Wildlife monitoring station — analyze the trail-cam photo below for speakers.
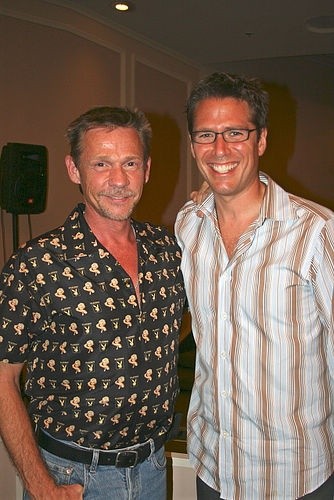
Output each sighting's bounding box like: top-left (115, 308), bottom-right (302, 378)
top-left (0, 143), bottom-right (48, 215)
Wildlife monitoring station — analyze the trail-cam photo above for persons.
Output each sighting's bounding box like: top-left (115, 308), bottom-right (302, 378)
top-left (0, 103), bottom-right (214, 500)
top-left (173, 73), bottom-right (334, 499)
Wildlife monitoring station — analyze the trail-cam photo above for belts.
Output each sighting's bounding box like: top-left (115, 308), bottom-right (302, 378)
top-left (33, 415), bottom-right (175, 469)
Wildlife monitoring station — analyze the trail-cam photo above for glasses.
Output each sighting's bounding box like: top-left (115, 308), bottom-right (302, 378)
top-left (190, 127), bottom-right (264, 145)
